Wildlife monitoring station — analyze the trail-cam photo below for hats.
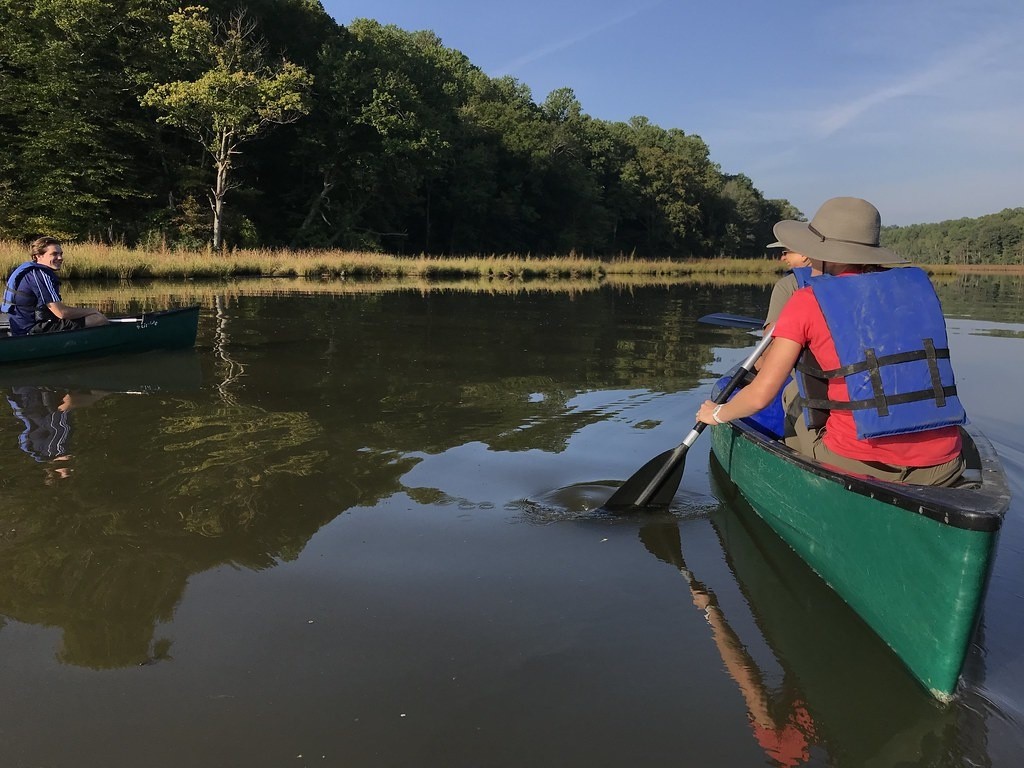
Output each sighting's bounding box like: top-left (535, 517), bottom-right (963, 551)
top-left (766, 239), bottom-right (785, 248)
top-left (773, 197), bottom-right (912, 265)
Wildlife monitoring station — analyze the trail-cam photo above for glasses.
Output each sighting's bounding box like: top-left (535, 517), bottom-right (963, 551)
top-left (782, 250), bottom-right (792, 256)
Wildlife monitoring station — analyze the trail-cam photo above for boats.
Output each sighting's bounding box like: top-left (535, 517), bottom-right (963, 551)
top-left (0, 304), bottom-right (200, 365)
top-left (715, 341), bottom-right (1013, 705)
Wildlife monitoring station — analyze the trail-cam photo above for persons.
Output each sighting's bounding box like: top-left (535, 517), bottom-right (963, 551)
top-left (4, 385), bottom-right (119, 496)
top-left (690, 579), bottom-right (836, 767)
top-left (696, 196), bottom-right (968, 488)
top-left (0, 236), bottom-right (111, 337)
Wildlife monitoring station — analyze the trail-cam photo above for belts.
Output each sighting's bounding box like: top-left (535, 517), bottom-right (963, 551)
top-left (861, 459), bottom-right (915, 474)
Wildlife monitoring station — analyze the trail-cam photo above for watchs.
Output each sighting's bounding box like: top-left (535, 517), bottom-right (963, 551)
top-left (712, 403), bottom-right (726, 423)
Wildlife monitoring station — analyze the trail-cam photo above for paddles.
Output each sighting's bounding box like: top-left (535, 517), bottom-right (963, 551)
top-left (600, 319), bottom-right (778, 512)
top-left (108, 312), bottom-right (147, 325)
